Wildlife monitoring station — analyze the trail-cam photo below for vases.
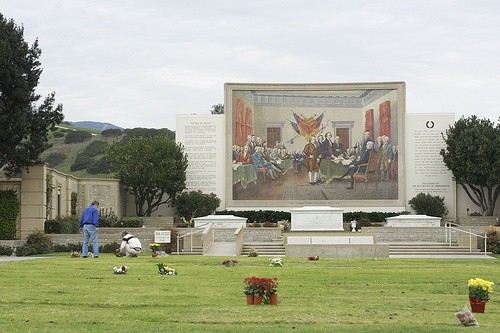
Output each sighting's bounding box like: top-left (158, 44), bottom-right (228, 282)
top-left (269, 294), bottom-right (277, 305)
top-left (152, 252), bottom-right (158, 257)
top-left (470, 299), bottom-right (485, 313)
top-left (246, 294), bottom-right (254, 304)
top-left (253, 294), bottom-right (262, 305)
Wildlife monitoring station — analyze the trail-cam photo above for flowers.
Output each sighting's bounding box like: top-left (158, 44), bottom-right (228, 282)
top-left (243, 276), bottom-right (278, 296)
top-left (222, 259), bottom-right (239, 267)
top-left (114, 266), bottom-right (127, 274)
top-left (269, 258), bottom-right (284, 267)
top-left (308, 255), bottom-right (320, 260)
top-left (468, 278), bottom-right (495, 302)
top-left (149, 244), bottom-right (160, 252)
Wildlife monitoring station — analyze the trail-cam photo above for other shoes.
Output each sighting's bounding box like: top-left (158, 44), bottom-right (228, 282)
top-left (82, 256), bottom-right (87, 258)
top-left (94, 256), bottom-right (98, 258)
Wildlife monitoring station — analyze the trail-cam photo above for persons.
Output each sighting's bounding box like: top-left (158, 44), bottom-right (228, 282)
top-left (79, 201), bottom-right (100, 258)
top-left (119, 231), bottom-right (142, 257)
